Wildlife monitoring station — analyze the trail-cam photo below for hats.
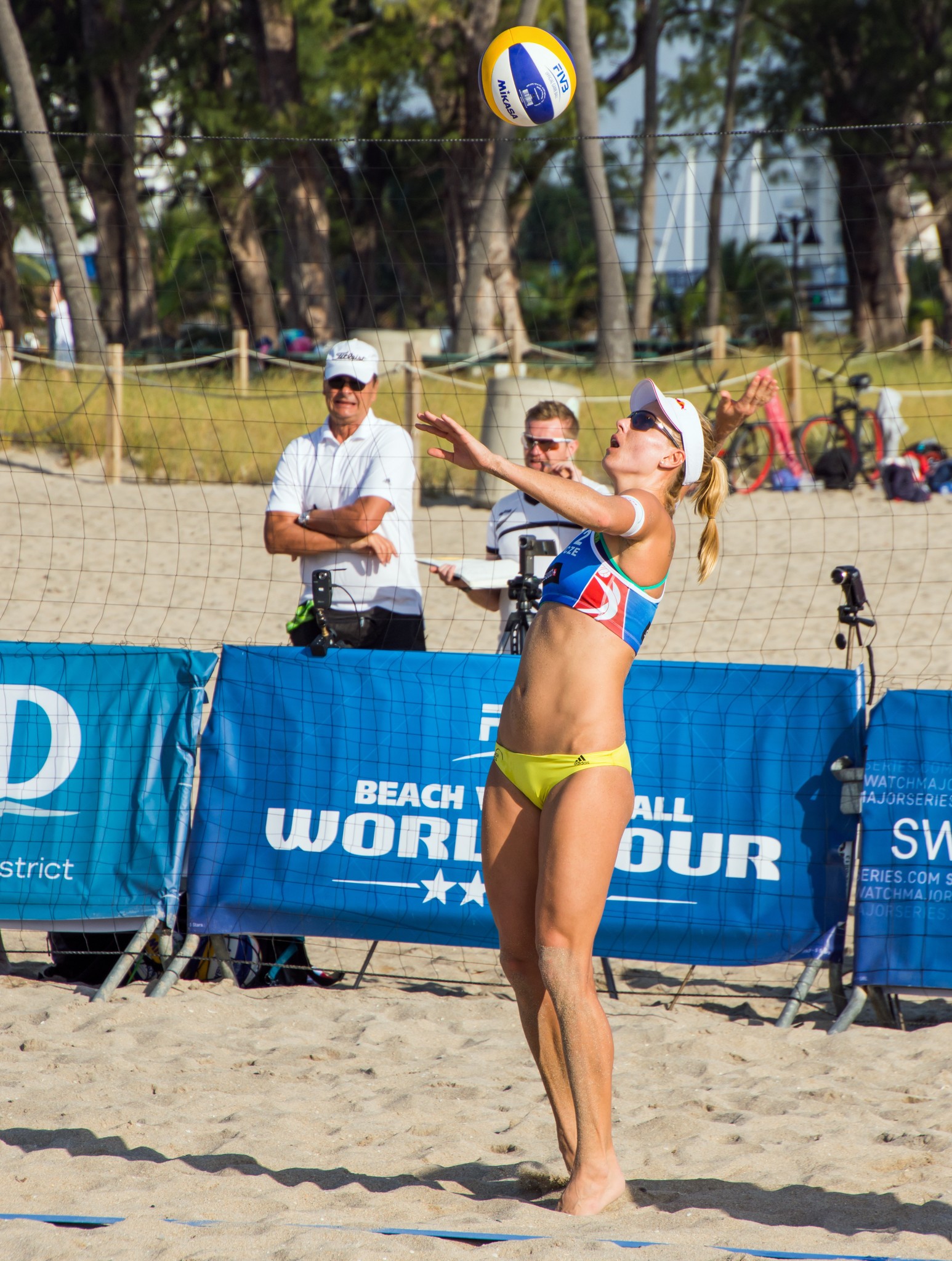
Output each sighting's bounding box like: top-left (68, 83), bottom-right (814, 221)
top-left (324, 338), bottom-right (379, 384)
top-left (630, 378), bottom-right (704, 486)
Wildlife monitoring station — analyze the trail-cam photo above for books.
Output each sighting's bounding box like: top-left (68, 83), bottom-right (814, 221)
top-left (414, 556), bottom-right (523, 591)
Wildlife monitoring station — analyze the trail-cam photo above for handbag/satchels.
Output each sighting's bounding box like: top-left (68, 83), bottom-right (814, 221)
top-left (880, 456), bottom-right (932, 503)
top-left (814, 447), bottom-right (856, 490)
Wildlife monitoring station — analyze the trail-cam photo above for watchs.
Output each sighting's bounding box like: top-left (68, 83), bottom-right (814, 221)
top-left (297, 502), bottom-right (318, 528)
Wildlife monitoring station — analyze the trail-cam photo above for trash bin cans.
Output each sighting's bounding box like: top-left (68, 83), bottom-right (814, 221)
top-left (473, 377), bottom-right (582, 510)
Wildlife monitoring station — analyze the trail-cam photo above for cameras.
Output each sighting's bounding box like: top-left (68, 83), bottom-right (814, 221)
top-left (312, 569), bottom-right (332, 610)
top-left (832, 566), bottom-right (866, 606)
top-left (518, 534), bottom-right (558, 577)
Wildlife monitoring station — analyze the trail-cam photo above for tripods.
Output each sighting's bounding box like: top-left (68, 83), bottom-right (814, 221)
top-left (497, 611), bottom-right (535, 658)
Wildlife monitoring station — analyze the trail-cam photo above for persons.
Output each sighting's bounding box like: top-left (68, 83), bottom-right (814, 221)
top-left (262, 338), bottom-right (425, 651)
top-left (431, 401), bottom-right (612, 654)
top-left (415, 371), bottom-right (781, 1207)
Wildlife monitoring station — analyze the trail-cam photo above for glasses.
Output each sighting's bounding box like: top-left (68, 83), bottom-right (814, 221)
top-left (626, 410), bottom-right (684, 469)
top-left (329, 376), bottom-right (366, 391)
top-left (521, 433), bottom-right (574, 452)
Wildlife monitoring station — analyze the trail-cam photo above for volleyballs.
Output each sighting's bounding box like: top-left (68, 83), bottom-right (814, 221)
top-left (477, 25), bottom-right (578, 128)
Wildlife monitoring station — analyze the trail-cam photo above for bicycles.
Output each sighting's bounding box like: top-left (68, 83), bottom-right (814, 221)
top-left (701, 344), bottom-right (893, 494)
